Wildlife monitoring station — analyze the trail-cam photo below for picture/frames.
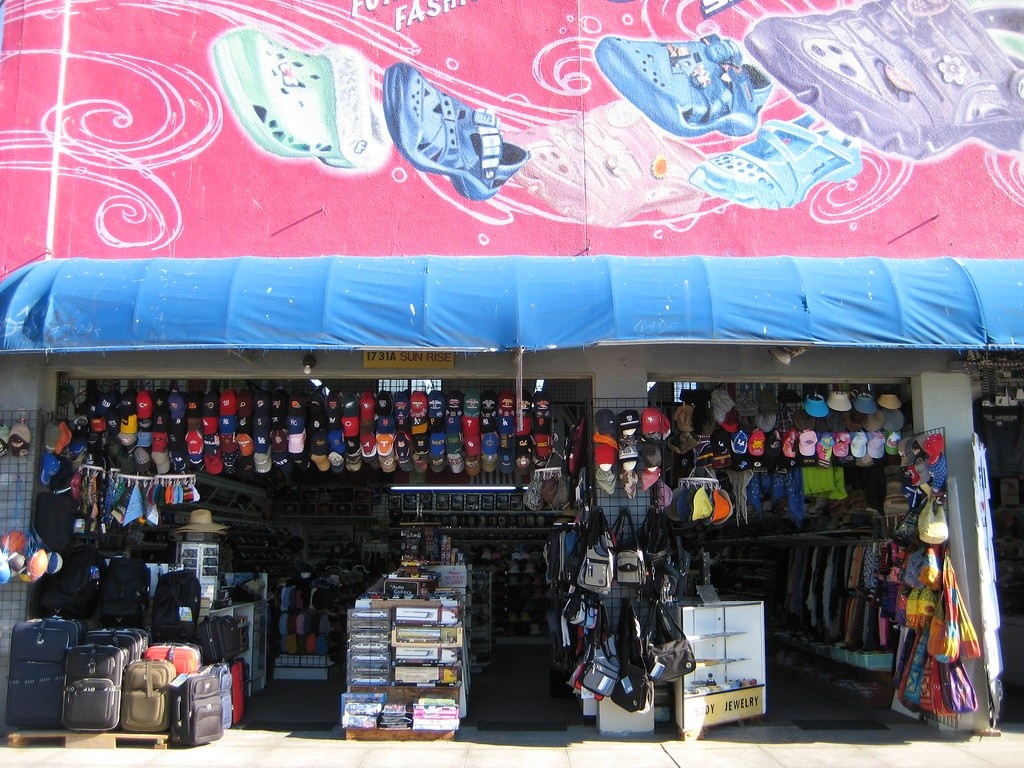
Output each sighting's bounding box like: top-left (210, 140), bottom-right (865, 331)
top-left (335, 503), bottom-right (353, 516)
top-left (281, 488), bottom-right (319, 515)
top-left (466, 494), bottom-right (479, 511)
top-left (510, 494), bottom-right (524, 511)
top-left (353, 488), bottom-right (371, 503)
top-left (388, 494), bottom-right (403, 510)
top-left (481, 494), bottom-right (495, 511)
top-left (436, 494), bottom-right (449, 511)
top-left (420, 494), bottom-right (433, 510)
top-left (451, 495), bottom-right (465, 510)
top-left (403, 494), bottom-right (417, 511)
top-left (496, 495), bottom-right (509, 511)
top-left (336, 488), bottom-right (353, 503)
top-left (354, 503), bottom-right (371, 516)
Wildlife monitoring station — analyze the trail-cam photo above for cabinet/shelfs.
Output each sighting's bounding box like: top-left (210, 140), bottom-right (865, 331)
top-left (675, 601), bottom-right (766, 728)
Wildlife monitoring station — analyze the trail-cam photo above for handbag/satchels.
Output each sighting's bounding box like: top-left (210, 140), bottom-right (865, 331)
top-left (883, 494), bottom-right (983, 715)
top-left (33, 544), bottom-right (104, 617)
top-left (150, 568), bottom-right (202, 641)
top-left (543, 507), bottom-right (698, 712)
top-left (100, 555), bottom-right (151, 627)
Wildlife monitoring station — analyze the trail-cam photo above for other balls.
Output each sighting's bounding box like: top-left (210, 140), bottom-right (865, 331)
top-left (44, 552), bottom-right (64, 574)
top-left (0, 557), bottom-right (11, 583)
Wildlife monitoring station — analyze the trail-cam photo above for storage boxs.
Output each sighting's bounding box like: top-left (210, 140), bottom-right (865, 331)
top-left (830, 647), bottom-right (839, 659)
top-left (813, 646), bottom-right (833, 654)
top-left (846, 650), bottom-right (859, 665)
top-left (857, 652), bottom-right (893, 669)
top-left (838, 648), bottom-right (849, 663)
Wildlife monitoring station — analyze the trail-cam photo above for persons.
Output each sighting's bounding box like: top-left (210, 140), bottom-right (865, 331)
top-left (280, 536), bottom-right (317, 610)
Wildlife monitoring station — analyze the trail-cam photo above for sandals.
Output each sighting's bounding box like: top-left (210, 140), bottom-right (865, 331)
top-left (384, 63), bottom-right (531, 200)
top-left (690, 112), bottom-right (864, 211)
top-left (745, 0), bottom-right (1024, 160)
top-left (597, 32), bottom-right (774, 137)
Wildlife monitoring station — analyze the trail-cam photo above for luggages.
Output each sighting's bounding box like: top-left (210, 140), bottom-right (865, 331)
top-left (4, 615), bottom-right (83, 727)
top-left (197, 610), bottom-right (242, 663)
top-left (62, 625), bottom-right (250, 747)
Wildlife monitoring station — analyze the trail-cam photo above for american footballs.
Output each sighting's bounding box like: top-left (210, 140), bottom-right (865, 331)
top-left (27, 549), bottom-right (49, 581)
top-left (8, 551), bottom-right (27, 574)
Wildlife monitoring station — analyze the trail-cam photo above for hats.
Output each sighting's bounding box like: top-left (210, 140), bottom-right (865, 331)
top-left (272, 559), bottom-right (371, 664)
top-left (473, 550), bottom-right (544, 637)
top-left (0, 383), bottom-right (947, 533)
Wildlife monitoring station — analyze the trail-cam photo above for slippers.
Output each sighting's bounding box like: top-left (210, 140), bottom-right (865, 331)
top-left (502, 95), bottom-right (707, 225)
top-left (210, 25), bottom-right (393, 170)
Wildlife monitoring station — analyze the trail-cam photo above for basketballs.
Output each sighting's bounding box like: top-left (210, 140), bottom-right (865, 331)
top-left (1, 530), bottom-right (26, 553)
